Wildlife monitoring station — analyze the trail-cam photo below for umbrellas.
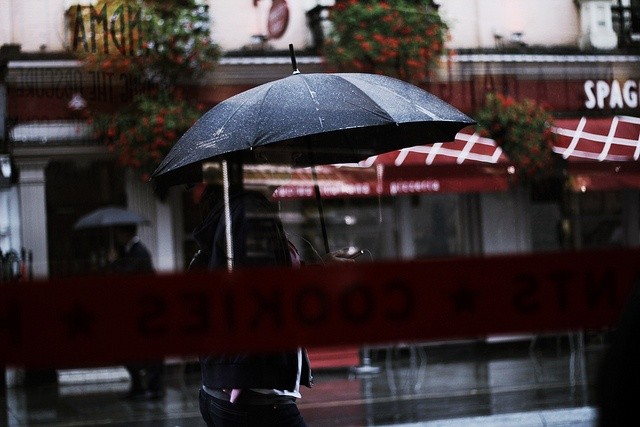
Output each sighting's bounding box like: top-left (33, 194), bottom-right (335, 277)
top-left (150, 44), bottom-right (478, 255)
top-left (72, 207), bottom-right (151, 229)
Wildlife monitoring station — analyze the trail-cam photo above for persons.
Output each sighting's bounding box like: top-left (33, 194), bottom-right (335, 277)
top-left (199, 193), bottom-right (355, 426)
top-left (107, 223), bottom-right (165, 399)
